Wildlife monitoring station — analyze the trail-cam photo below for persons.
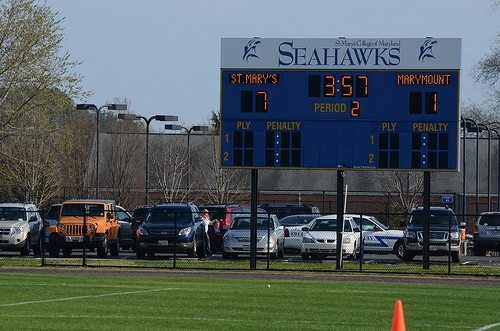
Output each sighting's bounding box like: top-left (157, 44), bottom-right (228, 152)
top-left (198, 209), bottom-right (214, 259)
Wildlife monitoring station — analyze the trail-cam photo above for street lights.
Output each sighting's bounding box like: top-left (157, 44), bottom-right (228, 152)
top-left (164, 124), bottom-right (209, 206)
top-left (75, 103), bottom-right (128, 199)
top-left (117, 114), bottom-right (178, 206)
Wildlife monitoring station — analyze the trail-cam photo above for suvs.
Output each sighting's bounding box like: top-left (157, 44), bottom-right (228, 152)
top-left (48, 199), bottom-right (122, 256)
top-left (304, 214), bottom-right (406, 259)
top-left (0, 203), bottom-right (43, 256)
top-left (473, 211), bottom-right (500, 257)
top-left (134, 202), bottom-right (205, 260)
top-left (400, 204), bottom-right (462, 263)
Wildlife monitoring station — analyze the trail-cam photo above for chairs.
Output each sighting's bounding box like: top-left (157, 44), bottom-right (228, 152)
top-left (262, 221), bottom-right (272, 226)
top-left (239, 221), bottom-right (249, 226)
top-left (318, 223), bottom-right (332, 230)
top-left (89, 207), bottom-right (98, 214)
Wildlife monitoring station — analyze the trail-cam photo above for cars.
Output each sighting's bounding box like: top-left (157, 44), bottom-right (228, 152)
top-left (279, 215), bottom-right (321, 249)
top-left (115, 205), bottom-right (134, 250)
top-left (301, 219), bottom-right (364, 260)
top-left (41, 204), bottom-right (62, 252)
top-left (223, 214), bottom-right (285, 260)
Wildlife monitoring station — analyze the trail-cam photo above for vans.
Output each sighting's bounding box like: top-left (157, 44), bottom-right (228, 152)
top-left (198, 204), bottom-right (270, 247)
